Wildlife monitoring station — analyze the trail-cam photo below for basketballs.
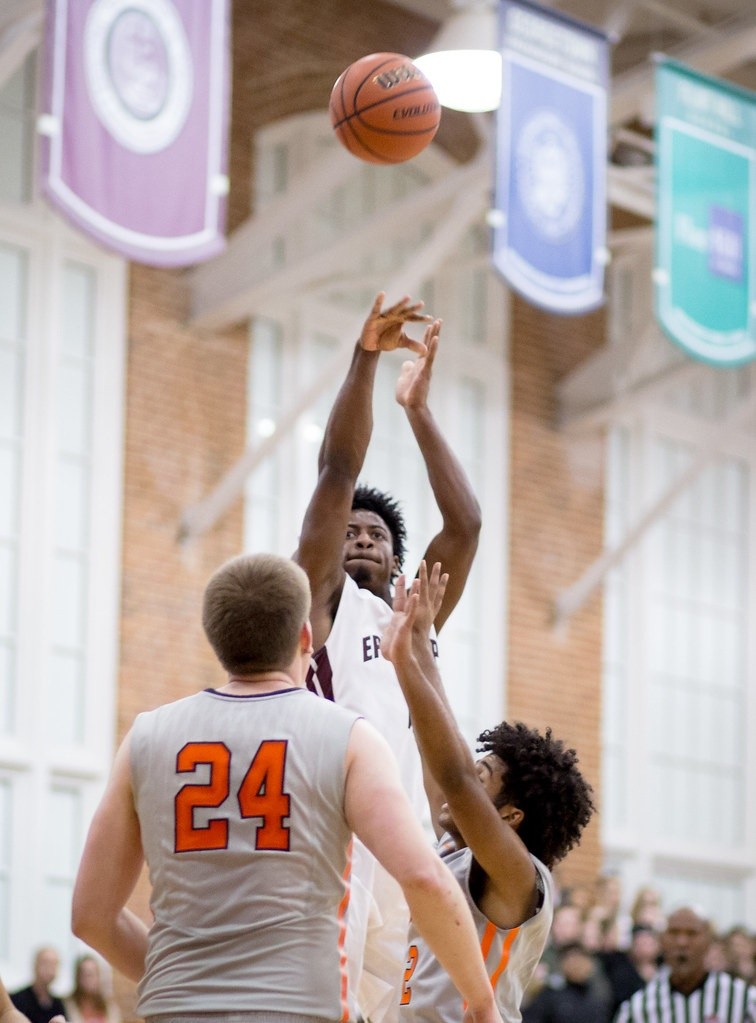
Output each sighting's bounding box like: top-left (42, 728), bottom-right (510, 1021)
top-left (328, 49), bottom-right (441, 166)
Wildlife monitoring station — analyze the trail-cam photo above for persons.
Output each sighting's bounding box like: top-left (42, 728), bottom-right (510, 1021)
top-left (71, 552), bottom-right (505, 1022)
top-left (384, 558), bottom-right (591, 1022)
top-left (2, 947), bottom-right (122, 1023)
top-left (288, 292), bottom-right (482, 1023)
top-left (523, 884), bottom-right (756, 1022)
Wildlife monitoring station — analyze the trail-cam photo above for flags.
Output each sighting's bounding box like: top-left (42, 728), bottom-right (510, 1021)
top-left (40, 1), bottom-right (234, 270)
top-left (486, 1), bottom-right (756, 367)
